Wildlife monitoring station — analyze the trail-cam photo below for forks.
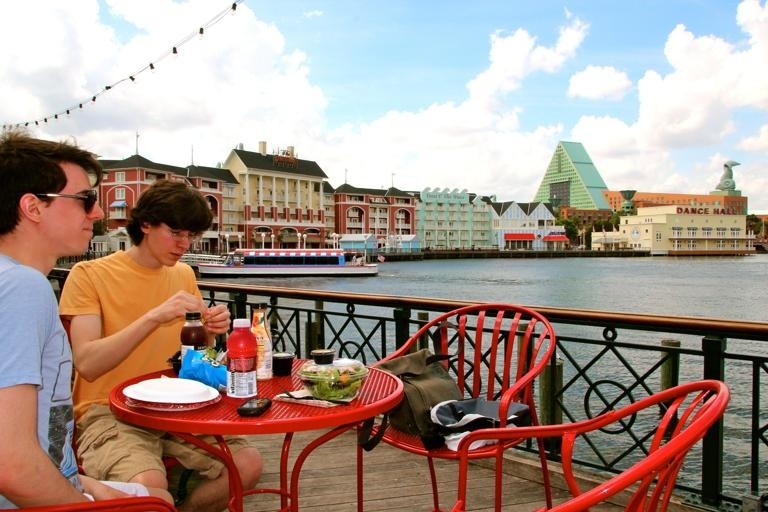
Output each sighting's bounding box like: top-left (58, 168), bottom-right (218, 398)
top-left (282, 388), bottom-right (351, 406)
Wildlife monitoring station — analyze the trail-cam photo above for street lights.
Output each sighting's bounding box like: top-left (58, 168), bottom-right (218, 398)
top-left (223, 232), bottom-right (342, 250)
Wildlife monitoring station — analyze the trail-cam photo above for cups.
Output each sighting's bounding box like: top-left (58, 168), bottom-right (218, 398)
top-left (272, 353), bottom-right (296, 376)
top-left (311, 349), bottom-right (335, 364)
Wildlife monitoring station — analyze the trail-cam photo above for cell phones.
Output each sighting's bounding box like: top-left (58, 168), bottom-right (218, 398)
top-left (237, 397), bottom-right (272, 416)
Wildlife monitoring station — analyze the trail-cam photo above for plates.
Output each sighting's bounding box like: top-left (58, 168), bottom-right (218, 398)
top-left (123, 378), bottom-right (222, 412)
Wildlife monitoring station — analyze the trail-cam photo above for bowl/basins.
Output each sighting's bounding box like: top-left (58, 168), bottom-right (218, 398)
top-left (296, 361), bottom-right (370, 398)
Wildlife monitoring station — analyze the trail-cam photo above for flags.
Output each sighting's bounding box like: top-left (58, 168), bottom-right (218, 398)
top-left (378, 254), bottom-right (385, 263)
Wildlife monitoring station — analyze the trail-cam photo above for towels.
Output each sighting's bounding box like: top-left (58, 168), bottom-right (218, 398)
top-left (273, 388), bottom-right (341, 408)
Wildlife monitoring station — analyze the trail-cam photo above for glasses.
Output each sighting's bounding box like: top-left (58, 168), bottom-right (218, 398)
top-left (36, 190), bottom-right (97, 214)
top-left (147, 227), bottom-right (203, 242)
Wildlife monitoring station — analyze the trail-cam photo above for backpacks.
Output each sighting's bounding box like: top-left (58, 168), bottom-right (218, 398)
top-left (427, 397), bottom-right (531, 451)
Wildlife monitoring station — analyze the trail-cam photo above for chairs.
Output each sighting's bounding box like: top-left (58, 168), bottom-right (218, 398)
top-left (450, 378), bottom-right (730, 512)
top-left (2, 498), bottom-right (176, 512)
top-left (58, 291), bottom-right (195, 505)
top-left (354, 303), bottom-right (557, 512)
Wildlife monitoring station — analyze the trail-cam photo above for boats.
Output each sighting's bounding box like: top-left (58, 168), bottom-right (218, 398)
top-left (196, 248), bottom-right (380, 278)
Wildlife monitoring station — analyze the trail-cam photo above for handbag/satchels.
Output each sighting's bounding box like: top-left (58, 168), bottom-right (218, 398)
top-left (358, 348), bottom-right (464, 448)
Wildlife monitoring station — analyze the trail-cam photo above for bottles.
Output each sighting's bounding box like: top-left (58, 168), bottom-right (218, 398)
top-left (226, 317), bottom-right (259, 401)
top-left (180, 311), bottom-right (206, 366)
top-left (250, 303), bottom-right (276, 381)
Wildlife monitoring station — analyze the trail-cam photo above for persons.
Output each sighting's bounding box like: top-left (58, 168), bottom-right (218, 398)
top-left (0, 134), bottom-right (175, 510)
top-left (57, 177), bottom-right (264, 511)
